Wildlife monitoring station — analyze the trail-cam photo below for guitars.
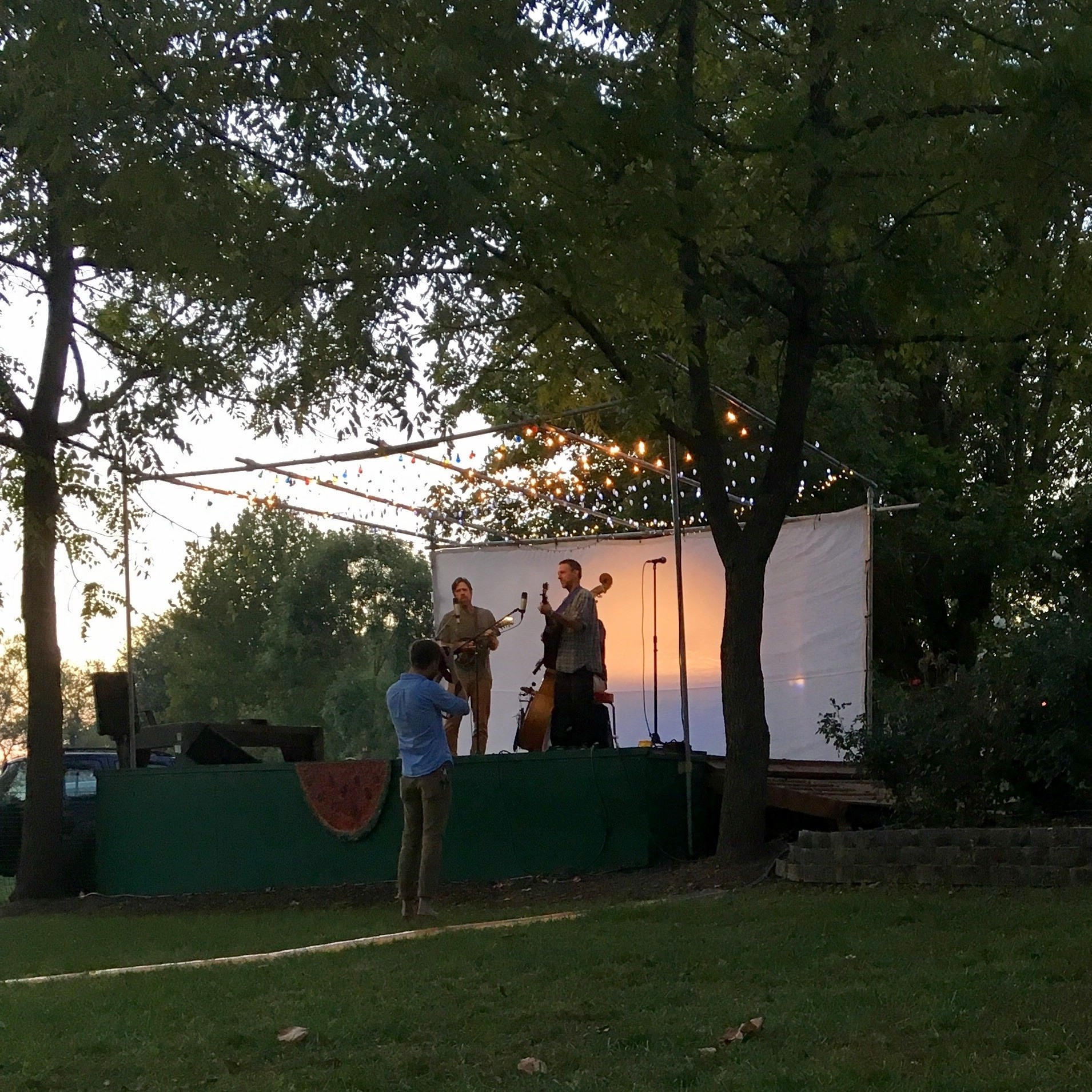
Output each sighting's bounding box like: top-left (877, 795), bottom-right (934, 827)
top-left (538, 581), bottom-right (565, 669)
top-left (454, 616), bottom-right (514, 668)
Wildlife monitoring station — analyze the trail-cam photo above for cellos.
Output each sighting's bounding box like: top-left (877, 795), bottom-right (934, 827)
top-left (517, 572), bottom-right (614, 753)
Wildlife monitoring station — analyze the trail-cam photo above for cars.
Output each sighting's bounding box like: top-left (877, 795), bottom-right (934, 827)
top-left (11, 781), bottom-right (99, 801)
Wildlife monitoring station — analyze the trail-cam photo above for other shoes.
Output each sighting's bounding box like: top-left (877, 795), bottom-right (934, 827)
top-left (418, 908), bottom-right (439, 916)
top-left (400, 910), bottom-right (413, 917)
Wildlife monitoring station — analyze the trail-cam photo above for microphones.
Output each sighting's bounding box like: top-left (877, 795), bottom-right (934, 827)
top-left (518, 592), bottom-right (528, 614)
top-left (452, 597), bottom-right (462, 624)
top-left (647, 557), bottom-right (667, 564)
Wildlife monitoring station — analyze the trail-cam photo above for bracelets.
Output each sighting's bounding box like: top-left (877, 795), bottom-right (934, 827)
top-left (546, 609), bottom-right (554, 617)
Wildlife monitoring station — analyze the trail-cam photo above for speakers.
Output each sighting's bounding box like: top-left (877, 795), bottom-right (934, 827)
top-left (185, 726), bottom-right (264, 764)
top-left (550, 705), bottom-right (613, 749)
top-left (92, 671), bottom-right (141, 735)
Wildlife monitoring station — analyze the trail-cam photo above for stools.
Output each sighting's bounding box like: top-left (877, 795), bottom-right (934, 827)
top-left (593, 692), bottom-right (619, 748)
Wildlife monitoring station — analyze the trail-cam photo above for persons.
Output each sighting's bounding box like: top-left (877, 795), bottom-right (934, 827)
top-left (434, 576), bottom-right (501, 758)
top-left (538, 559), bottom-right (603, 751)
top-left (385, 639), bottom-right (470, 917)
top-left (593, 620), bottom-right (608, 693)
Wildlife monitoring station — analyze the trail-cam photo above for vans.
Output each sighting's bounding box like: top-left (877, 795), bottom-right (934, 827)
top-left (0, 747), bottom-right (176, 878)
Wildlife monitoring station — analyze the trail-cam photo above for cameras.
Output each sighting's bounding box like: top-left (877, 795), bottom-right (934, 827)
top-left (437, 645), bottom-right (450, 671)
